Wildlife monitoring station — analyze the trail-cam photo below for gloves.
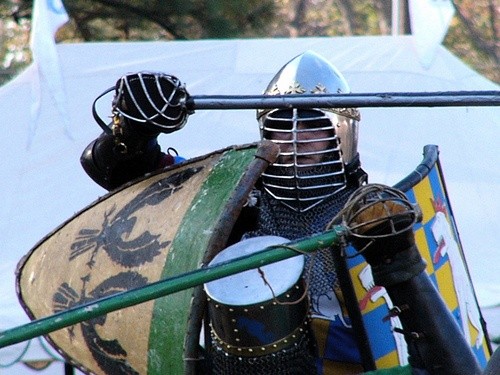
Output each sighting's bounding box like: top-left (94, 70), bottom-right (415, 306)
top-left (116, 78), bottom-right (188, 135)
top-left (345, 180), bottom-right (418, 255)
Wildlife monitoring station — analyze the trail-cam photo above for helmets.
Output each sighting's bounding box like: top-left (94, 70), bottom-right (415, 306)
top-left (256, 52), bottom-right (360, 212)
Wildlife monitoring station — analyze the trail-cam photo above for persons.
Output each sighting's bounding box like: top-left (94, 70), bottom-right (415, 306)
top-left (80, 53), bottom-right (374, 375)
top-left (341, 184), bottom-right (485, 375)
